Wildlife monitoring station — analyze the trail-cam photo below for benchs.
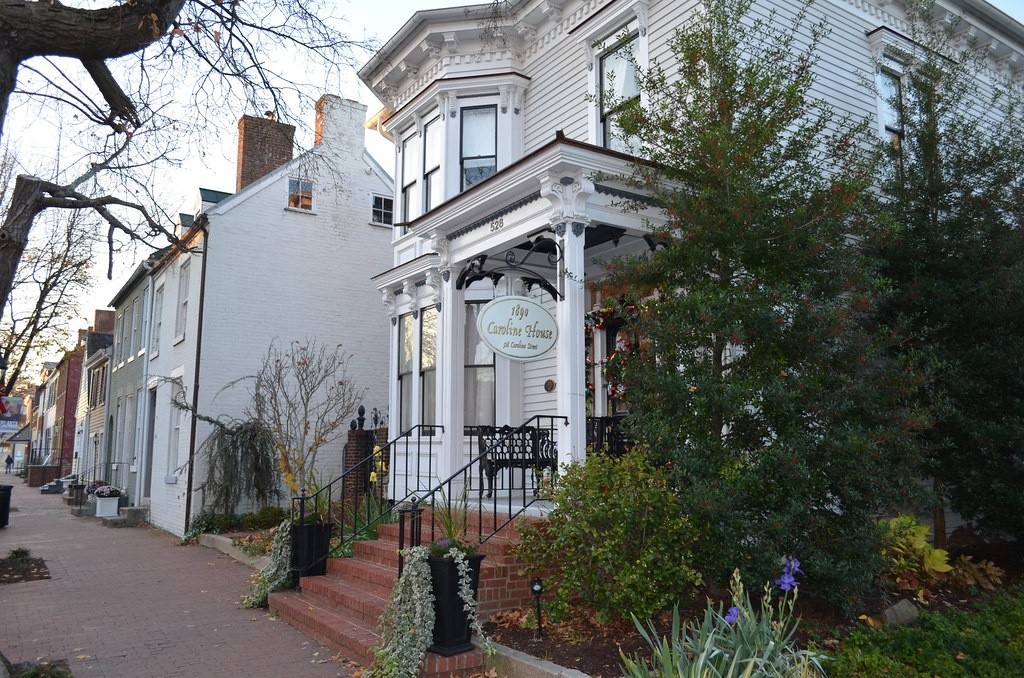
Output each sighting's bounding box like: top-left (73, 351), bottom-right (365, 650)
top-left (476, 425), bottom-right (558, 498)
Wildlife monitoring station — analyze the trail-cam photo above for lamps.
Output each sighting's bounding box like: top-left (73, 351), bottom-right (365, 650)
top-left (531, 577), bottom-right (543, 595)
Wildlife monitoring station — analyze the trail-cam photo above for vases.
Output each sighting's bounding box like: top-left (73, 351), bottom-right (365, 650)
top-left (95, 496), bottom-right (119, 518)
top-left (88, 494), bottom-right (97, 500)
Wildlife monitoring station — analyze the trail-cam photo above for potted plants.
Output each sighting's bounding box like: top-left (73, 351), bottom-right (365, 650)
top-left (242, 470), bottom-right (335, 608)
top-left (362, 476), bottom-right (497, 678)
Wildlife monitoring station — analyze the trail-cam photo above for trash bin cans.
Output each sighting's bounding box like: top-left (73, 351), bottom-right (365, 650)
top-left (0, 484), bottom-right (15, 530)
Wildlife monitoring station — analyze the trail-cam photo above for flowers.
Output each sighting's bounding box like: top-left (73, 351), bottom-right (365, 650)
top-left (303, 511), bottom-right (312, 523)
top-left (430, 536), bottom-right (454, 557)
top-left (87, 480), bottom-right (121, 498)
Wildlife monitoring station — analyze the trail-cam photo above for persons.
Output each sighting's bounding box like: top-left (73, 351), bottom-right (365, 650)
top-left (4, 455), bottom-right (14, 474)
